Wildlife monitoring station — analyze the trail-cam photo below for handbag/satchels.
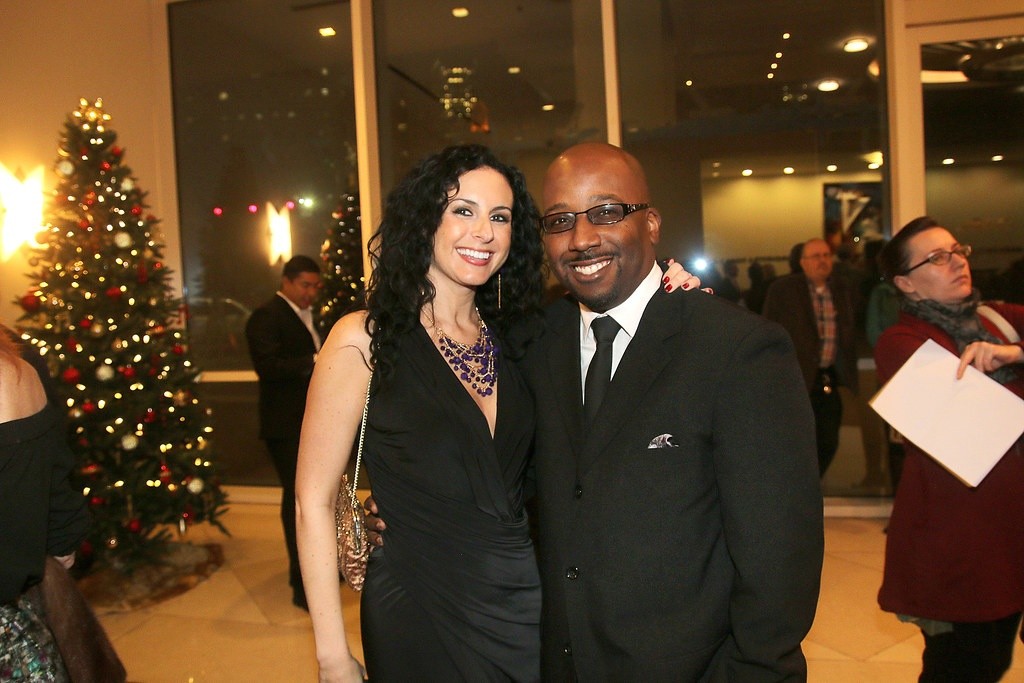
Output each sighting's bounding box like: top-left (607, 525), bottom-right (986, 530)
top-left (336, 476), bottom-right (370, 592)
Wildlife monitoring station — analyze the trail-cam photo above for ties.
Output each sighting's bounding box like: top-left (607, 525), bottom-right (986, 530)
top-left (582, 316), bottom-right (622, 432)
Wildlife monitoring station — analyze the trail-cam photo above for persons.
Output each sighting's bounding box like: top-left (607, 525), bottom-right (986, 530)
top-left (243, 257), bottom-right (351, 618)
top-left (296, 138), bottom-right (719, 682)
top-left (657, 238), bottom-right (893, 327)
top-left (764, 235), bottom-right (854, 478)
top-left (0, 332), bottom-right (122, 681)
top-left (877, 218), bottom-right (1024, 683)
top-left (356, 138), bottom-right (825, 682)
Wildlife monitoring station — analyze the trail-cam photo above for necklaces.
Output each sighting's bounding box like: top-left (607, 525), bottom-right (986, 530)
top-left (420, 300), bottom-right (500, 398)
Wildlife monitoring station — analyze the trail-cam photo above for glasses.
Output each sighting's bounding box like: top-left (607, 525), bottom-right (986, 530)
top-left (903, 244), bottom-right (972, 276)
top-left (805, 254), bottom-right (833, 260)
top-left (539, 203), bottom-right (652, 234)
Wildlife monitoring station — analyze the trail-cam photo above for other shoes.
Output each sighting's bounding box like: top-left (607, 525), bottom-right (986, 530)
top-left (292, 590), bottom-right (308, 612)
